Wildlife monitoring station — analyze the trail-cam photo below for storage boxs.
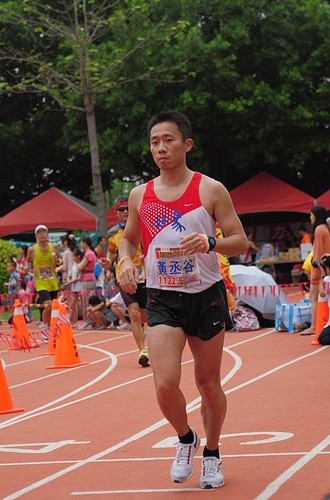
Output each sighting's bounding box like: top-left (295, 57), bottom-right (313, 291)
top-left (280, 251), bottom-right (289, 260)
top-left (288, 248), bottom-right (300, 259)
top-left (272, 270), bottom-right (302, 277)
top-left (300, 243), bottom-right (312, 259)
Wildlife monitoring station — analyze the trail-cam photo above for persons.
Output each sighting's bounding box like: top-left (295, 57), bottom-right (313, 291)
top-left (117, 109), bottom-right (250, 489)
top-left (316, 253), bottom-right (330, 346)
top-left (24, 224), bottom-right (63, 331)
top-left (99, 196), bottom-right (151, 368)
top-left (297, 225), bottom-right (311, 245)
top-left (235, 229), bottom-right (261, 263)
top-left (297, 206), bottom-right (330, 335)
top-left (3, 235), bottom-right (131, 331)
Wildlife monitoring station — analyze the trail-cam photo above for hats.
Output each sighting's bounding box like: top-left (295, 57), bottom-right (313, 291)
top-left (34, 225), bottom-right (49, 235)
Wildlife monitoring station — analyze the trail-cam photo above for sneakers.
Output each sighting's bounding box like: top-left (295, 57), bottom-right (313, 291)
top-left (198, 452), bottom-right (224, 490)
top-left (138, 350), bottom-right (150, 367)
top-left (171, 426), bottom-right (201, 483)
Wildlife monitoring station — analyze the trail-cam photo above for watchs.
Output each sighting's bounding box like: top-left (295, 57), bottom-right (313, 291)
top-left (206, 236), bottom-right (216, 255)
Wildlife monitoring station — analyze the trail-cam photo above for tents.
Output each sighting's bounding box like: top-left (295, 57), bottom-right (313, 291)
top-left (317, 190), bottom-right (330, 210)
top-left (0, 187), bottom-right (118, 235)
top-left (229, 171), bottom-right (317, 215)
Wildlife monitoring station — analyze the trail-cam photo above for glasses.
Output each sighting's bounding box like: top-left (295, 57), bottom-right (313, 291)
top-left (116, 207), bottom-right (129, 212)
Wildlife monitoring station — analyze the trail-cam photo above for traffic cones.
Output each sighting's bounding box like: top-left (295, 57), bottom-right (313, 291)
top-left (41, 299), bottom-right (61, 355)
top-left (44, 303), bottom-right (89, 369)
top-left (0, 356), bottom-right (25, 415)
top-left (6, 298), bottom-right (40, 350)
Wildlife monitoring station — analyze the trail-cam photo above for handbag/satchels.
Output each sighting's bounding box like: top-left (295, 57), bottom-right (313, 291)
top-left (275, 299), bottom-right (312, 332)
top-left (233, 305), bottom-right (260, 332)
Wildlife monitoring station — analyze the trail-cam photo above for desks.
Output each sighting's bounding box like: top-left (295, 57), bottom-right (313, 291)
top-left (253, 258), bottom-right (307, 282)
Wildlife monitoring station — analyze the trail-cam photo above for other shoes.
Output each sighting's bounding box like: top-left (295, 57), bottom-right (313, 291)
top-left (298, 328), bottom-right (315, 334)
top-left (105, 325), bottom-right (115, 330)
top-left (116, 323), bottom-right (128, 330)
top-left (97, 325), bottom-right (106, 330)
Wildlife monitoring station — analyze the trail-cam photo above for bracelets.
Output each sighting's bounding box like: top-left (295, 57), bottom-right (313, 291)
top-left (118, 256), bottom-right (132, 268)
top-left (56, 259), bottom-right (62, 265)
